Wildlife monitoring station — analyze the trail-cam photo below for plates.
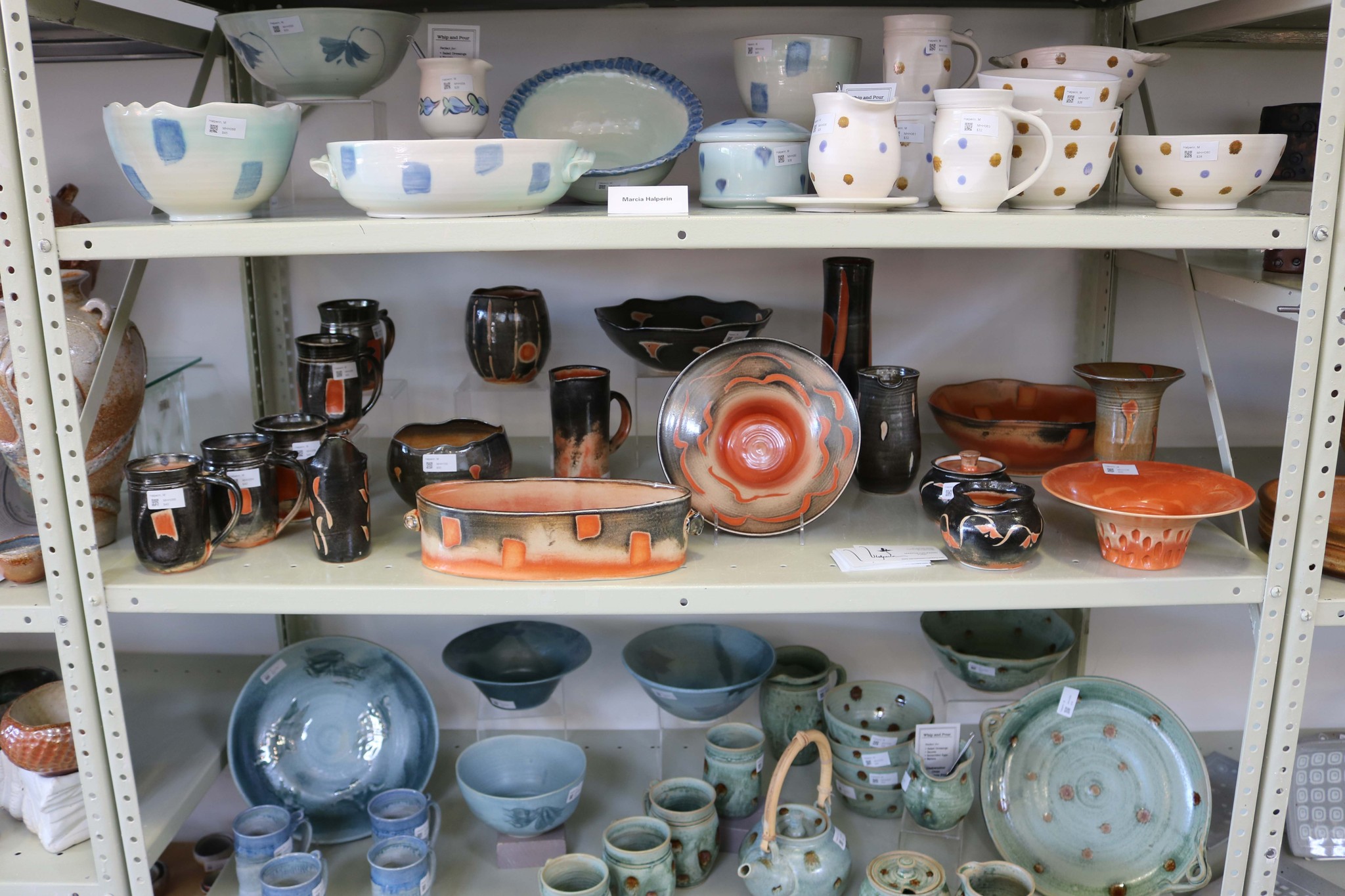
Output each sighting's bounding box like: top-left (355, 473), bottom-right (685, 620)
top-left (978, 676), bottom-right (1345, 896)
top-left (227, 634), bottom-right (440, 845)
top-left (656, 337), bottom-right (862, 540)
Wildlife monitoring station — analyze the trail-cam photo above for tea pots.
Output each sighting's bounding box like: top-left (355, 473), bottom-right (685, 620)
top-left (735, 728), bottom-right (854, 895)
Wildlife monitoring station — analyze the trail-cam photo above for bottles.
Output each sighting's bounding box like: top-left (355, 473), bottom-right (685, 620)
top-left (0, 269), bottom-right (148, 549)
top-left (859, 851), bottom-right (949, 896)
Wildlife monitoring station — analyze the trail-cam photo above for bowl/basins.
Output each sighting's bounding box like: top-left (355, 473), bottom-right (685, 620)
top-left (0, 667), bottom-right (78, 778)
top-left (592, 297), bottom-right (774, 375)
top-left (920, 609), bottom-right (1078, 693)
top-left (974, 45), bottom-right (1289, 211)
top-left (441, 618), bottom-right (592, 839)
top-left (918, 379), bottom-right (1345, 578)
top-left (101, 8), bottom-right (865, 221)
top-left (621, 625), bottom-right (934, 821)
top-left (387, 418), bottom-right (705, 584)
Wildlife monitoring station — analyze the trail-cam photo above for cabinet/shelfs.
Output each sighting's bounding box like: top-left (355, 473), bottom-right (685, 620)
top-left (0, 0), bottom-right (1345, 896)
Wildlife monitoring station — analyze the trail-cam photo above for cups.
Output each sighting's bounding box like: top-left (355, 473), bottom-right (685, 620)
top-left (954, 860), bottom-right (1037, 896)
top-left (0, 534), bottom-right (47, 586)
top-left (539, 644), bottom-right (846, 896)
top-left (146, 787), bottom-right (440, 896)
top-left (466, 285), bottom-right (632, 479)
top-left (808, 12), bottom-right (1052, 213)
top-left (417, 58), bottom-right (493, 141)
top-left (1072, 361), bottom-right (1186, 463)
top-left (124, 298), bottom-right (396, 577)
top-left (821, 256), bottom-right (922, 495)
